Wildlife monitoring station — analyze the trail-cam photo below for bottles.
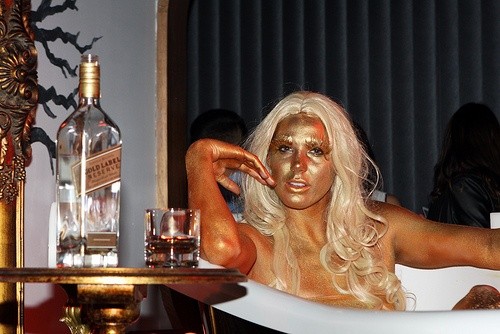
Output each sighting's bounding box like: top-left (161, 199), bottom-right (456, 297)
top-left (55, 54), bottom-right (123, 270)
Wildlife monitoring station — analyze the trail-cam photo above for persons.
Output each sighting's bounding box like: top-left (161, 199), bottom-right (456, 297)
top-left (185, 92), bottom-right (500, 313)
top-left (189, 103), bottom-right (500, 229)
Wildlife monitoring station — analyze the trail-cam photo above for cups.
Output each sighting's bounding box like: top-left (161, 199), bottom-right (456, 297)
top-left (142, 209), bottom-right (202, 267)
top-left (48, 201), bottom-right (80, 268)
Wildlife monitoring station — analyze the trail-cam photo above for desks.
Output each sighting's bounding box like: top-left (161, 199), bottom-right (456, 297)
top-left (0, 268), bottom-right (248, 334)
